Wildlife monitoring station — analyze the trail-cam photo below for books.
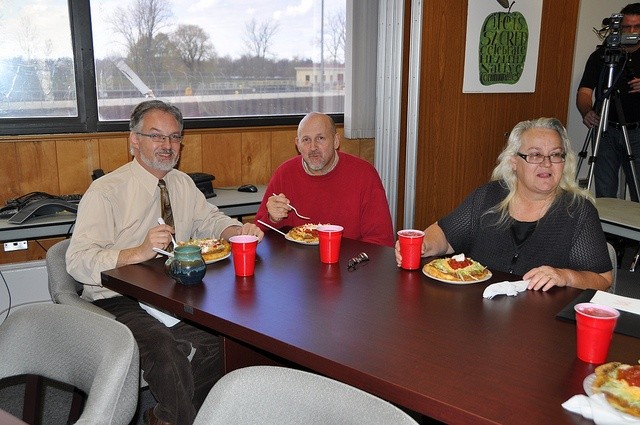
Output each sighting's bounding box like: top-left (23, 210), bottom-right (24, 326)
top-left (555, 288), bottom-right (639, 338)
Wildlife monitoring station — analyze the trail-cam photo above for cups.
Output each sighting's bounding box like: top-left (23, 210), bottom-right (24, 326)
top-left (574, 303), bottom-right (620, 364)
top-left (316, 224), bottom-right (344, 263)
top-left (165, 245), bottom-right (206, 287)
top-left (229, 235), bottom-right (258, 277)
top-left (397, 229), bottom-right (425, 270)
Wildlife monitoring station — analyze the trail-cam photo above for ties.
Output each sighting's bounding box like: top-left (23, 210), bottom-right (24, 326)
top-left (157, 179), bottom-right (176, 256)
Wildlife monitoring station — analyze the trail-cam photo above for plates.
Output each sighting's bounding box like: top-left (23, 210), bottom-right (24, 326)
top-left (422, 259), bottom-right (493, 285)
top-left (583, 374), bottom-right (639, 425)
top-left (285, 233), bottom-right (319, 245)
top-left (168, 250), bottom-right (232, 265)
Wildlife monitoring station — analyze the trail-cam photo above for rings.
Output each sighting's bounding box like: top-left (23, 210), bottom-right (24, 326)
top-left (545, 272), bottom-right (551, 279)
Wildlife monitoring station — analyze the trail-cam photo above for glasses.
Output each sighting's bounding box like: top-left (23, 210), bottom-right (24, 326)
top-left (516, 152), bottom-right (567, 164)
top-left (136, 132), bottom-right (183, 143)
top-left (347, 252), bottom-right (370, 270)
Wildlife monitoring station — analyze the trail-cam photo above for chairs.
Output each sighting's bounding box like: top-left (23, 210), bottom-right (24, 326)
top-left (0, 302), bottom-right (142, 425)
top-left (585, 197), bottom-right (638, 249)
top-left (193, 364), bottom-right (423, 424)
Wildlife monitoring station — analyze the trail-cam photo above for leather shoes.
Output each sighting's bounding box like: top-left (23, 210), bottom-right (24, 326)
top-left (614, 242), bottom-right (624, 269)
top-left (142, 407), bottom-right (170, 424)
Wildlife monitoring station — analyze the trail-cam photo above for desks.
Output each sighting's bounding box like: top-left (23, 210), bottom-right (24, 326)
top-left (0, 185), bottom-right (269, 246)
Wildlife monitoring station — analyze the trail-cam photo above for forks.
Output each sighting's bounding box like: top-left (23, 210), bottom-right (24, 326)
top-left (273, 193), bottom-right (310, 220)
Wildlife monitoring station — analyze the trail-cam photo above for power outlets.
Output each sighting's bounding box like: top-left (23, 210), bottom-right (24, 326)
top-left (3, 240), bottom-right (27, 251)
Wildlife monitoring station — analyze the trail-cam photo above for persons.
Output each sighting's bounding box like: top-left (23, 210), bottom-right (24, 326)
top-left (65, 100), bottom-right (264, 423)
top-left (576, 3), bottom-right (640, 202)
top-left (395, 118), bottom-right (614, 292)
top-left (254, 111), bottom-right (394, 249)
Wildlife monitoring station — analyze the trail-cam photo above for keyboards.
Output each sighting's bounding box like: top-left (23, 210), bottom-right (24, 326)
top-left (6, 193), bottom-right (86, 208)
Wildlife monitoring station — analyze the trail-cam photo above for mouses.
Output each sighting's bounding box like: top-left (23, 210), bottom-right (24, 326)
top-left (238, 184), bottom-right (258, 193)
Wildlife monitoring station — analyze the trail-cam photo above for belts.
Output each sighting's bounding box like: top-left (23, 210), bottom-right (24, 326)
top-left (608, 122), bottom-right (639, 131)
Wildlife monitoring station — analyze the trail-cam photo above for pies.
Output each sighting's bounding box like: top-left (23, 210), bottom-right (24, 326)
top-left (289, 223), bottom-right (322, 244)
top-left (593, 360), bottom-right (639, 416)
top-left (177, 240), bottom-right (230, 261)
top-left (424, 253), bottom-right (486, 281)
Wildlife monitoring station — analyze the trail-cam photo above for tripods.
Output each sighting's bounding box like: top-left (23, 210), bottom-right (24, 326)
top-left (575, 61), bottom-right (640, 199)
